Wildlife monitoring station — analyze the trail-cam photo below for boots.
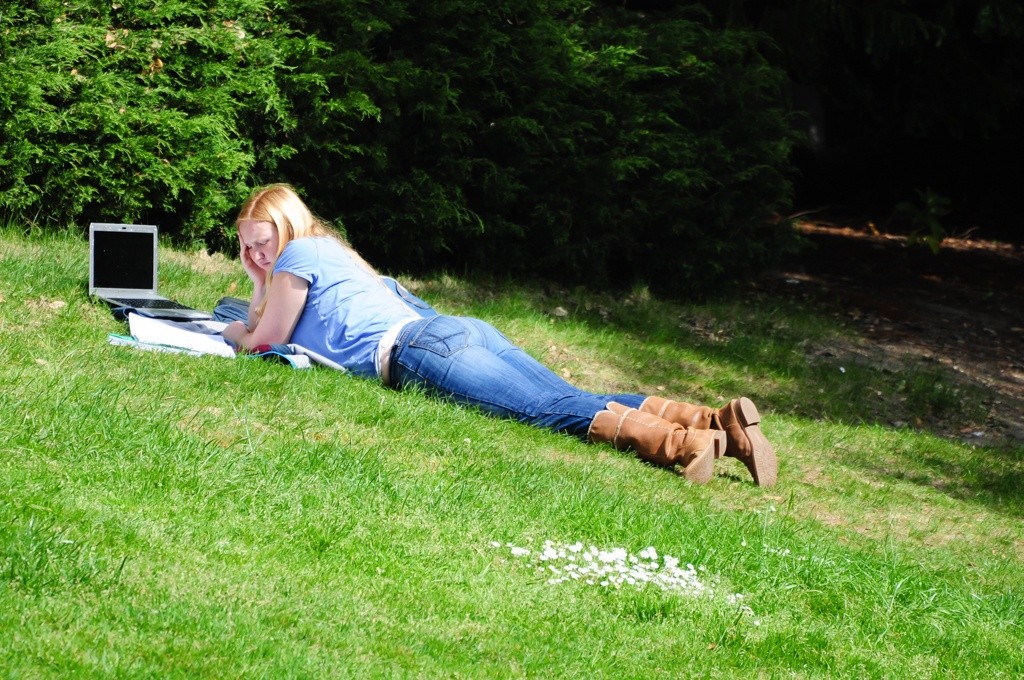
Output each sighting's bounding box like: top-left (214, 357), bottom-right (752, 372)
top-left (587, 400), bottom-right (728, 484)
top-left (639, 395), bottom-right (779, 489)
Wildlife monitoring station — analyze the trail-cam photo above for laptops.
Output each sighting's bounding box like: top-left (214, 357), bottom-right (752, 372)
top-left (89, 222), bottom-right (212, 319)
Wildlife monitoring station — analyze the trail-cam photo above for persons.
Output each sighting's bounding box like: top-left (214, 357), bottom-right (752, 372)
top-left (221, 186), bottom-right (777, 490)
